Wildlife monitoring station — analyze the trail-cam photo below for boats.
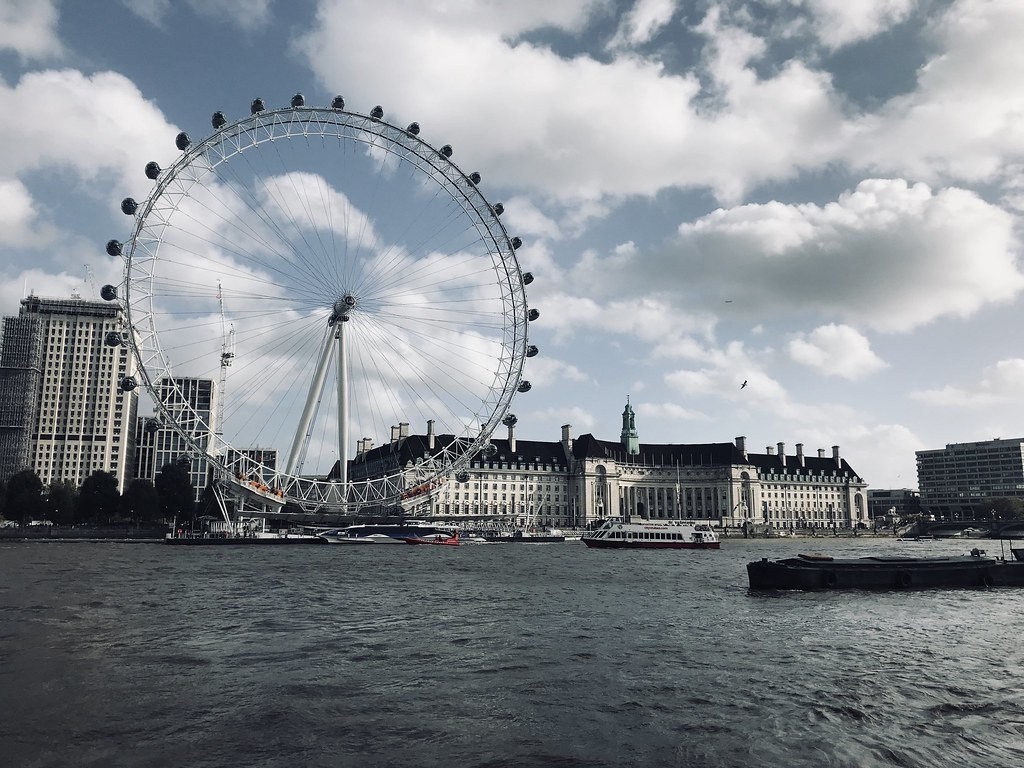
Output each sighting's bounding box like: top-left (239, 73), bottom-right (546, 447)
top-left (393, 529), bottom-right (460, 545)
top-left (480, 490), bottom-right (567, 542)
top-left (580, 515), bottom-right (722, 549)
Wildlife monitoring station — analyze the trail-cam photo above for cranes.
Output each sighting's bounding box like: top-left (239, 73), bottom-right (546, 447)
top-left (213, 282), bottom-right (236, 452)
